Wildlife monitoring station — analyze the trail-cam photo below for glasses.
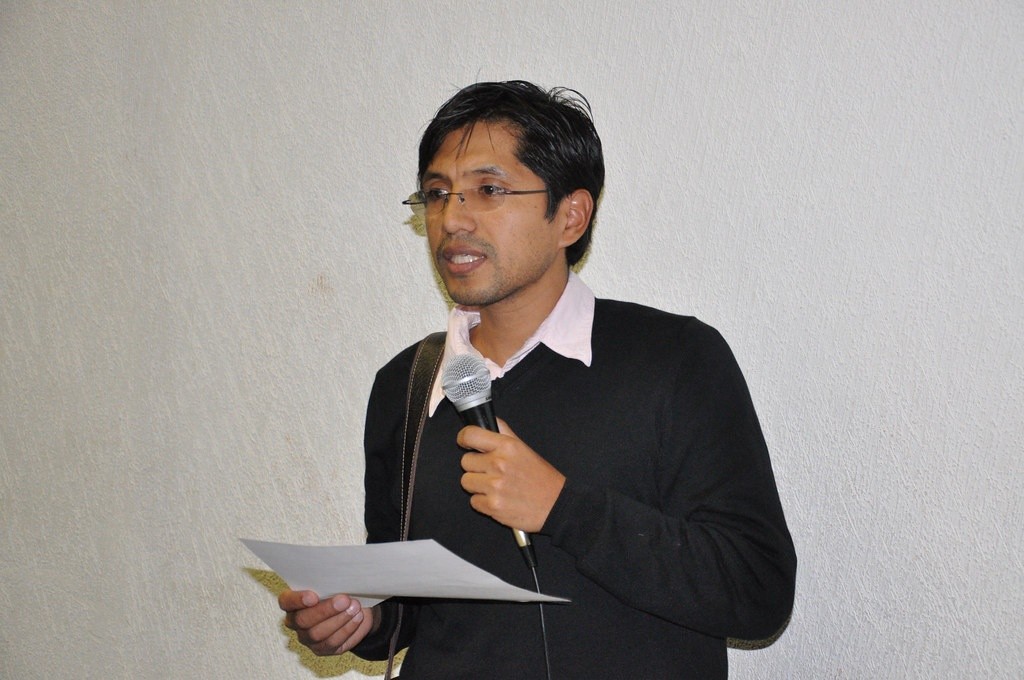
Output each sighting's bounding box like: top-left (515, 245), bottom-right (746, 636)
top-left (401, 184), bottom-right (549, 215)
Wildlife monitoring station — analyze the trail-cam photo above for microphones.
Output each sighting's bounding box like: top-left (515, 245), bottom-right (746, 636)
top-left (441, 353), bottom-right (539, 570)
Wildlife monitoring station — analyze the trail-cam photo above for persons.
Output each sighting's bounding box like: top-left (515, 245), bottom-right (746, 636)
top-left (276, 80), bottom-right (799, 680)
